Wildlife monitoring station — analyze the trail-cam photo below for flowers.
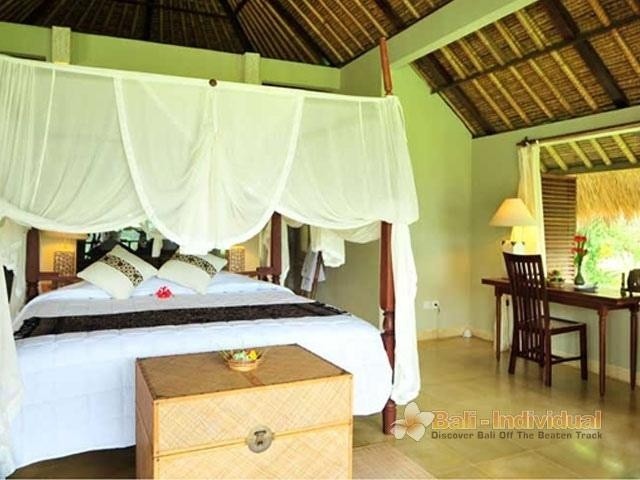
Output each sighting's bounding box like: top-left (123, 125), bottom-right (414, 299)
top-left (571, 232), bottom-right (590, 264)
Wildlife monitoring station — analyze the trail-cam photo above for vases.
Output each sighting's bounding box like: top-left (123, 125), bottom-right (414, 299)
top-left (574, 264), bottom-right (585, 285)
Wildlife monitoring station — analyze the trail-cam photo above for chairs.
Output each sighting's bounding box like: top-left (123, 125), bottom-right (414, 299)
top-left (503, 252), bottom-right (588, 387)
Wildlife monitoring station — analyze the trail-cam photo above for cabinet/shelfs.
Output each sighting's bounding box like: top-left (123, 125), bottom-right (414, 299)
top-left (130, 344), bottom-right (353, 480)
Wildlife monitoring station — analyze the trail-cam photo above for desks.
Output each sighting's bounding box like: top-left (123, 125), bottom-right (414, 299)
top-left (481, 277), bottom-right (640, 396)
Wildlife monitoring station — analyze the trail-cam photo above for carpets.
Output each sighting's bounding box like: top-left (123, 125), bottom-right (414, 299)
top-left (352, 441), bottom-right (437, 479)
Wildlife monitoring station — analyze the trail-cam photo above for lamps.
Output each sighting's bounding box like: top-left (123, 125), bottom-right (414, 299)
top-left (488, 198), bottom-right (537, 281)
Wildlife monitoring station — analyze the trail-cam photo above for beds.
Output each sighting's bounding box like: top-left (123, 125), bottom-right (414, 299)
top-left (0, 36), bottom-right (398, 479)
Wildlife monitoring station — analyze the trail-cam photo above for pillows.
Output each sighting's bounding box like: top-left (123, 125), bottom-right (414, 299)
top-left (74, 235), bottom-right (229, 301)
